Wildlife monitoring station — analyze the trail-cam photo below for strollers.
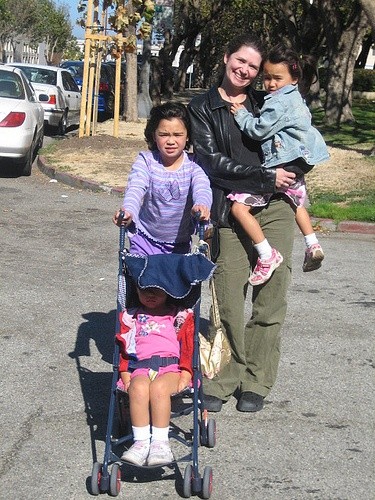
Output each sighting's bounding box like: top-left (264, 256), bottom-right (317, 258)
top-left (90, 211), bottom-right (217, 500)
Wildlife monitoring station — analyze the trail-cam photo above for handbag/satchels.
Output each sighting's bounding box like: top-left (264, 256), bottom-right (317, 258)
top-left (198, 331), bottom-right (232, 379)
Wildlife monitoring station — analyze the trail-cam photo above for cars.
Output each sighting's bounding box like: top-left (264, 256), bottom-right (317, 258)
top-left (4, 54), bottom-right (161, 135)
top-left (0, 65), bottom-right (50, 176)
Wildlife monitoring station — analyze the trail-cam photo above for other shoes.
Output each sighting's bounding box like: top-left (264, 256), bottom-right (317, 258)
top-left (238, 392), bottom-right (263, 412)
top-left (200, 395), bottom-right (222, 412)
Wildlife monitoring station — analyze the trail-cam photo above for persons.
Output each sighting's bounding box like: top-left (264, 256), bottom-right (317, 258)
top-left (111, 100), bottom-right (213, 258)
top-left (226, 49), bottom-right (331, 287)
top-left (116, 284), bottom-right (196, 471)
top-left (185, 31), bottom-right (299, 415)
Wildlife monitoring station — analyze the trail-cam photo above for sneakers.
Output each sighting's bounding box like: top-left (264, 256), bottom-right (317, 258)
top-left (121, 438), bottom-right (150, 466)
top-left (147, 439), bottom-right (175, 465)
top-left (302, 244), bottom-right (324, 272)
top-left (248, 249), bottom-right (283, 285)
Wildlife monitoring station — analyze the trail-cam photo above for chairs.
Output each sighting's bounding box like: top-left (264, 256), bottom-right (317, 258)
top-left (0, 81), bottom-right (17, 97)
